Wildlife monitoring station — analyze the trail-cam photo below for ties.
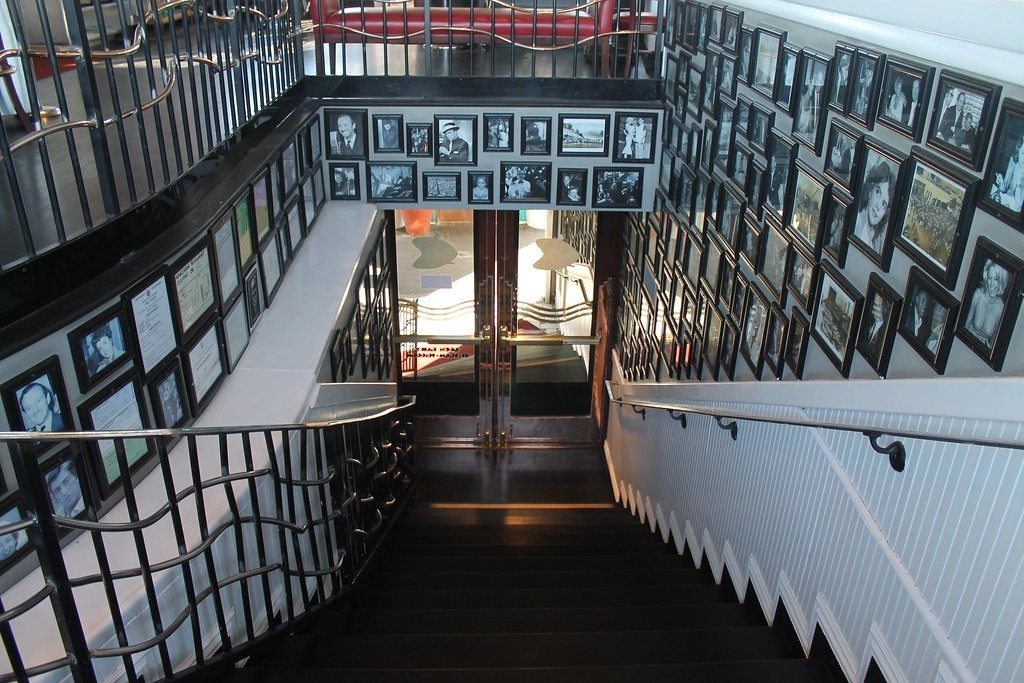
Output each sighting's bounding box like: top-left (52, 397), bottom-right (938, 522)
top-left (868, 323), bottom-right (876, 343)
top-left (449, 141), bottom-right (453, 153)
top-left (40, 426), bottom-right (46, 432)
top-left (346, 140), bottom-right (352, 151)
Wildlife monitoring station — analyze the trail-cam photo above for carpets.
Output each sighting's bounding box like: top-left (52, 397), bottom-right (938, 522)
top-left (402, 318), bottom-right (540, 375)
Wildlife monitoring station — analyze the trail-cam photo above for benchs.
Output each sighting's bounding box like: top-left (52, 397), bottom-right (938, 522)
top-left (311, 0), bottom-right (615, 78)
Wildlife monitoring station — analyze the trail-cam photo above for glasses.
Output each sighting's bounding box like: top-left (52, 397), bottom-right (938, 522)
top-left (949, 92), bottom-right (953, 97)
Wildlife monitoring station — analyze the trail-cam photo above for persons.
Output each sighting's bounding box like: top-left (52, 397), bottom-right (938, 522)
top-left (505, 165), bottom-right (546, 198)
top-left (747, 54), bottom-right (1023, 361)
top-left (0, 521), bottom-right (19, 560)
top-left (338, 113), bottom-right (362, 156)
top-left (335, 168), bottom-right (354, 195)
top-left (666, 5), bottom-right (737, 109)
top-left (473, 177), bottom-right (488, 199)
top-left (47, 462), bottom-right (85, 518)
top-left (160, 386), bottom-right (178, 427)
top-left (20, 383), bottom-right (64, 445)
top-left (91, 326), bottom-right (125, 372)
top-left (529, 124), bottom-right (541, 146)
top-left (370, 168), bottom-right (413, 198)
top-left (440, 123), bottom-right (468, 161)
top-left (412, 130), bottom-right (426, 152)
top-left (597, 178), bottom-right (638, 204)
top-left (562, 176), bottom-right (580, 201)
top-left (382, 120), bottom-right (397, 148)
top-left (488, 124), bottom-right (508, 147)
top-left (622, 117), bottom-right (647, 158)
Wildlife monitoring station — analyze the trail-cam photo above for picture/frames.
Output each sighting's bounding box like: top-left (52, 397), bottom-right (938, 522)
top-left (0, 0), bottom-right (1024, 575)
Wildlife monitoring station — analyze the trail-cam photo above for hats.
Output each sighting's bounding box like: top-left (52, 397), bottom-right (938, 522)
top-left (441, 122), bottom-right (460, 133)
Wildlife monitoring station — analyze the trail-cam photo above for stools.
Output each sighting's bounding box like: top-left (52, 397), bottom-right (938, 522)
top-left (610, 11), bottom-right (665, 78)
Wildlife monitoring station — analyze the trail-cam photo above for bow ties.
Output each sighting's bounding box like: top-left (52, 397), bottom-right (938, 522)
top-left (518, 180), bottom-right (524, 184)
